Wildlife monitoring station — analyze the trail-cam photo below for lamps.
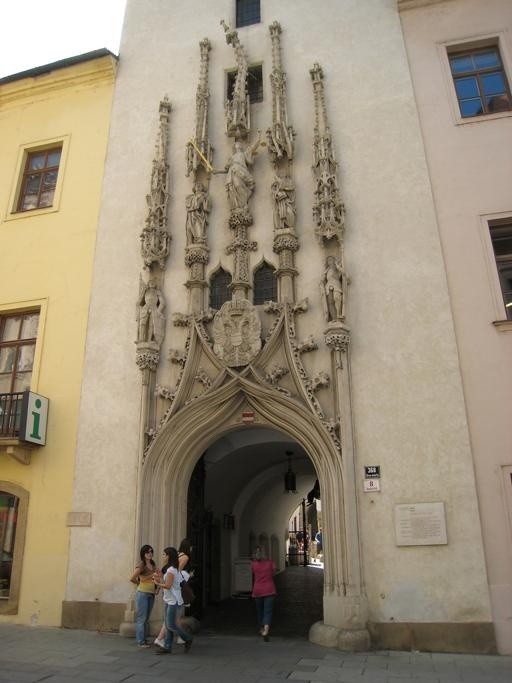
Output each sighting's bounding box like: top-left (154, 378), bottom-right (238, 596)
top-left (285, 451), bottom-right (297, 490)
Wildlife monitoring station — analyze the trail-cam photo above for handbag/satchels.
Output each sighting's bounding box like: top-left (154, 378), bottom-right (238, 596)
top-left (180, 580), bottom-right (197, 604)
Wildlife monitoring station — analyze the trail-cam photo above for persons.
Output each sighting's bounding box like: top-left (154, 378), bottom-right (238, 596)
top-left (129, 544), bottom-right (162, 648)
top-left (249, 543), bottom-right (278, 641)
top-left (134, 278), bottom-right (166, 344)
top-left (185, 182), bottom-right (209, 240)
top-left (318, 255), bottom-right (350, 321)
top-left (208, 127), bottom-right (263, 213)
top-left (295, 524), bottom-right (322, 559)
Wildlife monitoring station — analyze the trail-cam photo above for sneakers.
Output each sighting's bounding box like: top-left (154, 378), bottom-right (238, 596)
top-left (177, 637), bottom-right (193, 653)
top-left (137, 639), bottom-right (171, 653)
